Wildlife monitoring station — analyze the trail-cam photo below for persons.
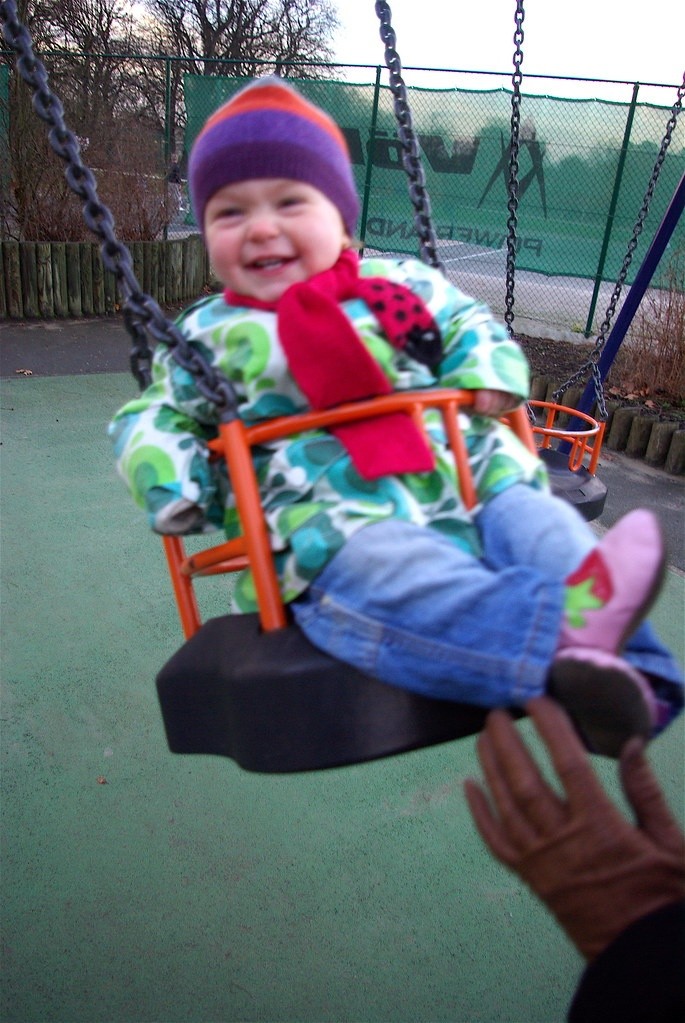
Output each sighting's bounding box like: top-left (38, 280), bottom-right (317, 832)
top-left (105, 73), bottom-right (685, 758)
top-left (463, 694), bottom-right (685, 1023)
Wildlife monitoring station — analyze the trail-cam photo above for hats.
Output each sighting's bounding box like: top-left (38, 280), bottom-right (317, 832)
top-left (188, 71), bottom-right (357, 243)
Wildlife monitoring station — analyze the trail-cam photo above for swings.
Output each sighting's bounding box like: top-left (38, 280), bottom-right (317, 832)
top-left (497, 0), bottom-right (685, 521)
top-left (0, 1), bottom-right (557, 776)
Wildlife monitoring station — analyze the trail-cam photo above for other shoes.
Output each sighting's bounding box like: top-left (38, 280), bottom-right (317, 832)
top-left (555, 508), bottom-right (668, 655)
top-left (543, 645), bottom-right (658, 761)
